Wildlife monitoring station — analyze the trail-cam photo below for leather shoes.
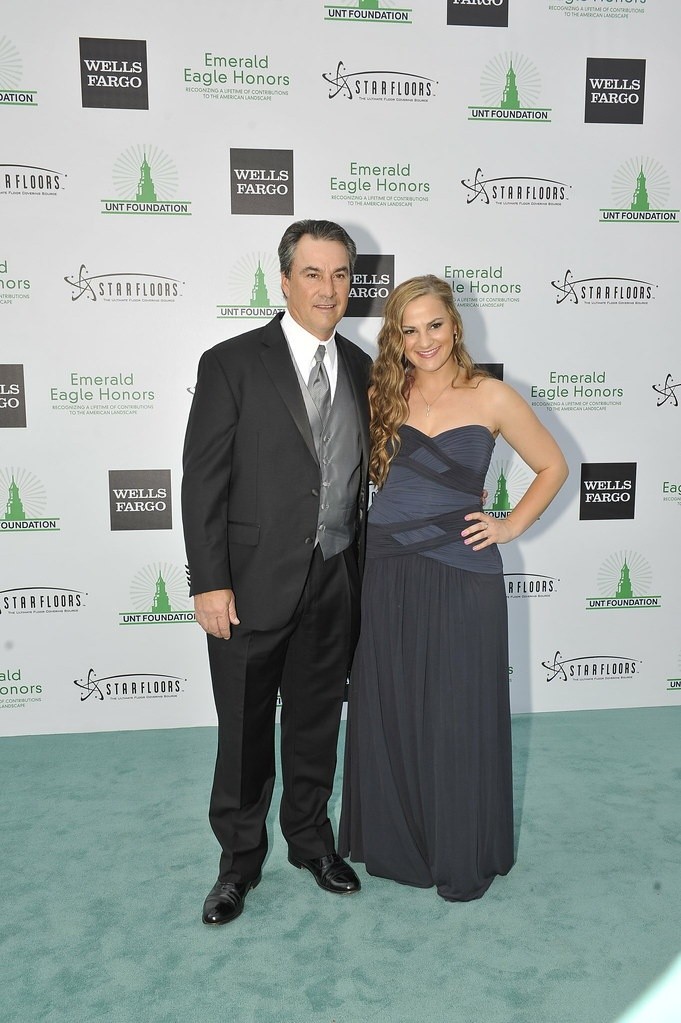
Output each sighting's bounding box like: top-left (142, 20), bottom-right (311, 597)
top-left (288, 852), bottom-right (362, 895)
top-left (201, 873), bottom-right (262, 924)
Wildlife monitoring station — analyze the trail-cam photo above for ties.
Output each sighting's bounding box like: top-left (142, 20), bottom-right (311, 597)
top-left (308, 345), bottom-right (331, 430)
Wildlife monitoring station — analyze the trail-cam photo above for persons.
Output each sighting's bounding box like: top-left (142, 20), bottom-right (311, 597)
top-left (338, 274), bottom-right (569, 904)
top-left (179, 220), bottom-right (375, 920)
